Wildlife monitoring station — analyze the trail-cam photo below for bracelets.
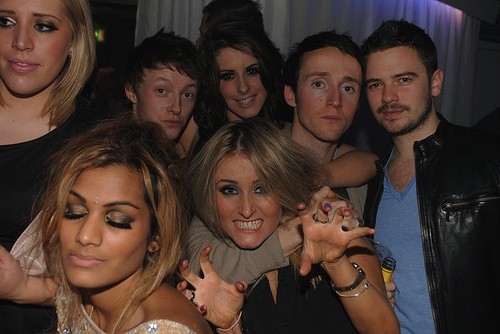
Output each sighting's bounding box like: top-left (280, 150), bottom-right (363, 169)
top-left (336, 282), bottom-right (369, 298)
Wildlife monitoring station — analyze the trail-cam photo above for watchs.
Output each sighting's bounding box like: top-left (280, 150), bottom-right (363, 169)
top-left (332, 261), bottom-right (367, 291)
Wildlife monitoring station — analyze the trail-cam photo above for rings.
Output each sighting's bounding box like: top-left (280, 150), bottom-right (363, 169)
top-left (190, 290), bottom-right (196, 302)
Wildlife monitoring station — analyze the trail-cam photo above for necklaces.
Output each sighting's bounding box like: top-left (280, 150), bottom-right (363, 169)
top-left (87, 304), bottom-right (96, 321)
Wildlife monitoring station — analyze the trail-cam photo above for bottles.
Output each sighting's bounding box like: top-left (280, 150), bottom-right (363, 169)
top-left (381, 256), bottom-right (396, 289)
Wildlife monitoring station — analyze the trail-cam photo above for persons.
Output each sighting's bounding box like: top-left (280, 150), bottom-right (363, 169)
top-left (172, 118), bottom-right (402, 334)
top-left (0, 1), bottom-right (98, 333)
top-left (185, 30), bottom-right (372, 285)
top-left (174, 0), bottom-right (377, 223)
top-left (124, 26), bottom-right (200, 144)
top-left (360, 19), bottom-right (500, 334)
top-left (39, 113), bottom-right (213, 333)
